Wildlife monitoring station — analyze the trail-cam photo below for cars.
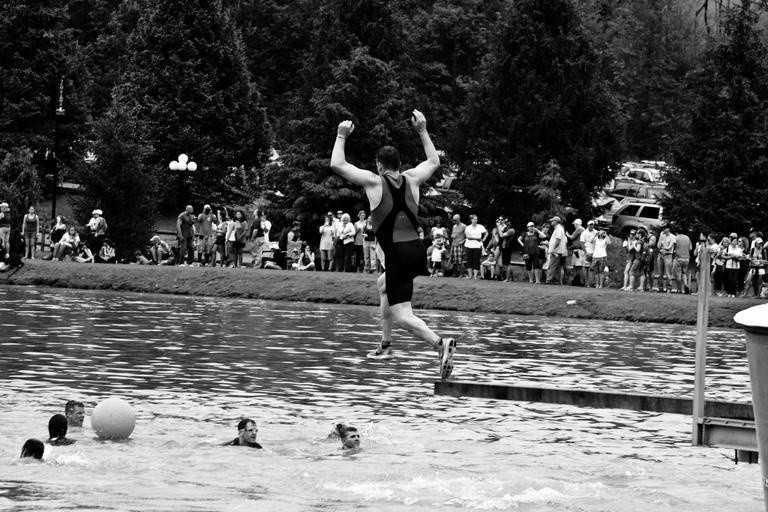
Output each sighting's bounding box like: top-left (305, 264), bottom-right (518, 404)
top-left (593, 158), bottom-right (681, 240)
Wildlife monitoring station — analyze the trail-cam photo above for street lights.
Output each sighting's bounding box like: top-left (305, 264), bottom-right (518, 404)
top-left (165, 153), bottom-right (199, 211)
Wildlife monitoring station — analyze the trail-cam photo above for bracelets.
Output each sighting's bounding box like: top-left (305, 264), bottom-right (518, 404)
top-left (37, 233), bottom-right (39, 235)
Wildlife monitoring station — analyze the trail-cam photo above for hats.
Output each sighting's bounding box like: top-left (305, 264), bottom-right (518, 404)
top-left (756, 237), bottom-right (763, 246)
top-left (731, 231), bottom-right (738, 239)
top-left (91, 209), bottom-right (103, 216)
top-left (0, 202), bottom-right (9, 208)
top-left (527, 221), bottom-right (535, 227)
top-left (573, 218), bottom-right (582, 226)
top-left (550, 215), bottom-right (562, 224)
top-left (587, 220), bottom-right (595, 228)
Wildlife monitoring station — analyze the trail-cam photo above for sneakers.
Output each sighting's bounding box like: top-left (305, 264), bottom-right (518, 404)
top-left (365, 340), bottom-right (395, 362)
top-left (619, 285), bottom-right (756, 298)
top-left (434, 335), bottom-right (458, 379)
top-left (429, 272), bottom-right (609, 288)
top-left (178, 262), bottom-right (242, 268)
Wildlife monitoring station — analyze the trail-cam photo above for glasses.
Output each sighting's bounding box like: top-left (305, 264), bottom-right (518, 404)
top-left (239, 428), bottom-right (255, 433)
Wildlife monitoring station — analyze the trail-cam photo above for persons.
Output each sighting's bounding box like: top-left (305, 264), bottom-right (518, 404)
top-left (44, 414), bottom-right (77, 446)
top-left (694, 225), bottom-right (767, 298)
top-left (1, 203), bottom-right (10, 260)
top-left (176, 205), bottom-right (272, 269)
top-left (43, 209), bottom-right (116, 264)
top-left (417, 214), bottom-right (515, 282)
top-left (263, 220), bottom-right (315, 271)
top-left (20, 439), bottom-right (44, 459)
top-left (131, 236), bottom-right (175, 265)
top-left (621, 225), bottom-right (692, 293)
top-left (64, 401), bottom-right (85, 426)
top-left (320, 209), bottom-right (384, 273)
top-left (518, 216), bottom-right (610, 289)
top-left (330, 109), bottom-right (457, 380)
top-left (338, 427), bottom-right (360, 450)
top-left (222, 419), bottom-right (262, 448)
top-left (21, 206), bottom-right (40, 259)
